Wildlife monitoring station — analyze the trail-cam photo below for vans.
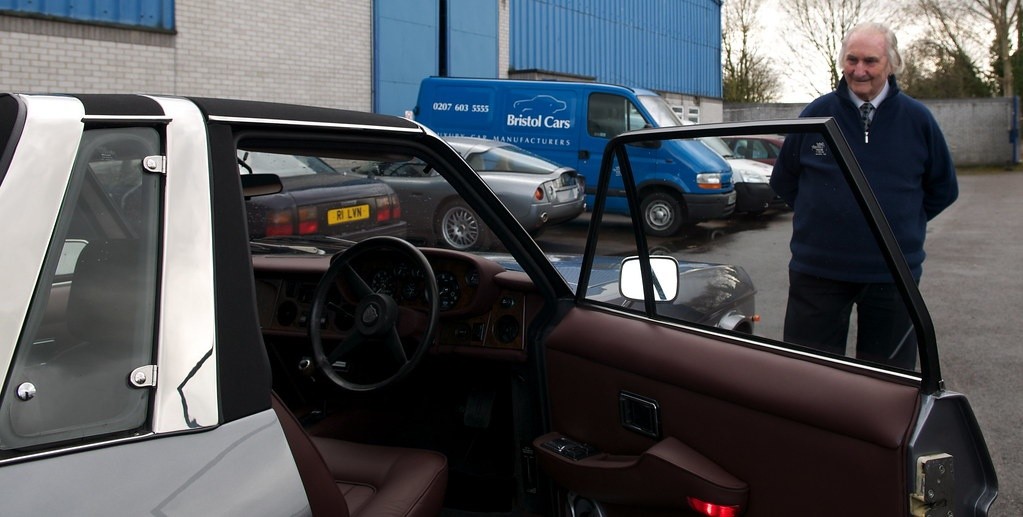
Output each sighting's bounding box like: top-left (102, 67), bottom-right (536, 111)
top-left (411, 75), bottom-right (740, 235)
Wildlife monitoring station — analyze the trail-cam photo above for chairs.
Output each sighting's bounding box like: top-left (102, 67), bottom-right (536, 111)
top-left (272, 390), bottom-right (451, 516)
top-left (40, 239), bottom-right (149, 372)
top-left (736, 144), bottom-right (746, 158)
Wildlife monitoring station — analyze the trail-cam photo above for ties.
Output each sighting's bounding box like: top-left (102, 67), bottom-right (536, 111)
top-left (860, 103), bottom-right (874, 131)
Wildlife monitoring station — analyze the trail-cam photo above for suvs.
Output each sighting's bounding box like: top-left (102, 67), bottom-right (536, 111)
top-left (629, 112), bottom-right (778, 224)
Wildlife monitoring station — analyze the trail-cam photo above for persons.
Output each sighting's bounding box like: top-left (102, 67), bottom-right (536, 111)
top-left (769, 22), bottom-right (960, 373)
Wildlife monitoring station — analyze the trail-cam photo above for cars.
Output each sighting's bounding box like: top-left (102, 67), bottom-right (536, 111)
top-left (713, 135), bottom-right (783, 166)
top-left (447, 251), bottom-right (763, 334)
top-left (2, 90), bottom-right (1002, 517)
top-left (86, 146), bottom-right (408, 252)
top-left (336, 136), bottom-right (588, 250)
